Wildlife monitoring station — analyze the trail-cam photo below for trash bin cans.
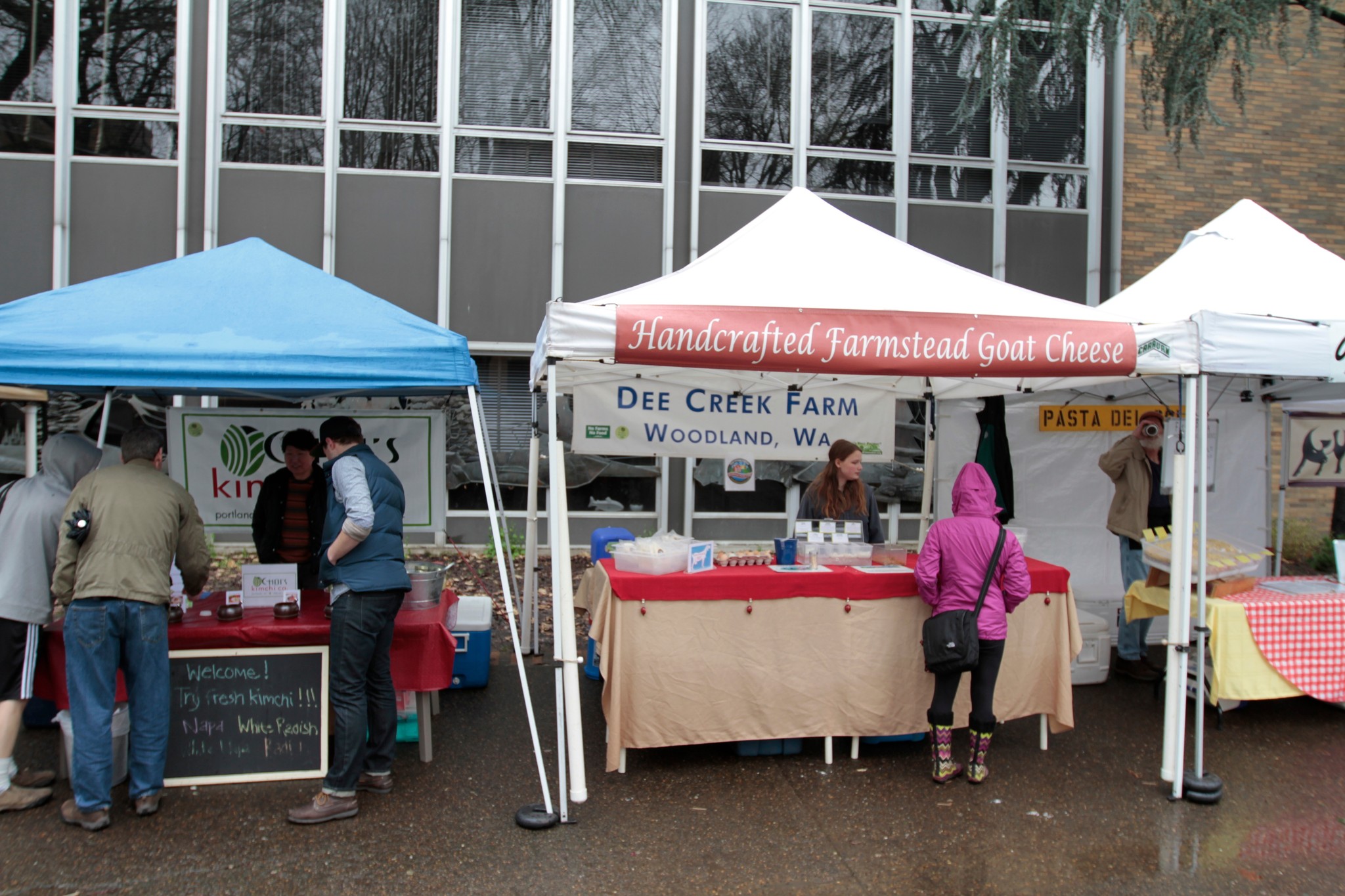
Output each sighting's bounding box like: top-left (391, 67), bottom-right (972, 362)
top-left (56, 703), bottom-right (129, 786)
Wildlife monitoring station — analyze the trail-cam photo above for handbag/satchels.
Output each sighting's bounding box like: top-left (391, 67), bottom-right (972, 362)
top-left (920, 608), bottom-right (979, 674)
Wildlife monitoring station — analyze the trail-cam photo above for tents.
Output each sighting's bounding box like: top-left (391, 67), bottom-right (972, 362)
top-left (930, 202), bottom-right (1344, 805)
top-left (494, 185), bottom-right (1199, 805)
top-left (0, 235), bottom-right (563, 831)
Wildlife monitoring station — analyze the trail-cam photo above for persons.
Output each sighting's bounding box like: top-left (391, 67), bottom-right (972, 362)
top-left (913, 462), bottom-right (1030, 784)
top-left (1, 434), bottom-right (107, 813)
top-left (287, 415), bottom-right (414, 825)
top-left (792, 438), bottom-right (886, 544)
top-left (48, 415), bottom-right (212, 836)
top-left (1097, 411), bottom-right (1173, 663)
top-left (250, 428), bottom-right (332, 589)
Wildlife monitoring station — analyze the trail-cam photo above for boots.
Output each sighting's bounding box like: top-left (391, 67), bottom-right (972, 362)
top-left (966, 712), bottom-right (997, 785)
top-left (928, 709), bottom-right (964, 784)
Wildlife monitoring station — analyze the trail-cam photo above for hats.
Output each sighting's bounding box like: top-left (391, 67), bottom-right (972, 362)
top-left (310, 416), bottom-right (361, 458)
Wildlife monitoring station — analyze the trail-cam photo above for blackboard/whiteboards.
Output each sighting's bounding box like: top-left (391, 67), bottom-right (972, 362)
top-left (162, 645), bottom-right (331, 782)
top-left (1158, 418), bottom-right (1220, 495)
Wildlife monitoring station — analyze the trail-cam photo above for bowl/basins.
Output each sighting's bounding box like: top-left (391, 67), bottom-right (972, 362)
top-left (324, 604), bottom-right (335, 619)
top-left (216, 603), bottom-right (242, 621)
top-left (168, 605), bottom-right (184, 624)
top-left (273, 602), bottom-right (300, 620)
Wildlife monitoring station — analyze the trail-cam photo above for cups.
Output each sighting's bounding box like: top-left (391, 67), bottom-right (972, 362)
top-left (1141, 424), bottom-right (1159, 439)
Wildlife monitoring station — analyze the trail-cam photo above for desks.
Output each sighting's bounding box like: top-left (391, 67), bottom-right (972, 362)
top-left (574, 550), bottom-right (1081, 778)
top-left (41, 574), bottom-right (462, 771)
top-left (1124, 576), bottom-right (1345, 729)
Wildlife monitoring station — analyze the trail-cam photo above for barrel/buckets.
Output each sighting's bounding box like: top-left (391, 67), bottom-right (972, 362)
top-left (395, 558), bottom-right (446, 610)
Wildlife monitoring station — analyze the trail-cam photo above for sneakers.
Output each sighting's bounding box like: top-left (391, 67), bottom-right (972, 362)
top-left (1142, 654), bottom-right (1165, 676)
top-left (135, 789), bottom-right (163, 815)
top-left (357, 773), bottom-right (393, 794)
top-left (61, 800), bottom-right (111, 831)
top-left (11, 767), bottom-right (56, 787)
top-left (1113, 655), bottom-right (1160, 682)
top-left (0, 783), bottom-right (55, 810)
top-left (287, 792), bottom-right (358, 823)
